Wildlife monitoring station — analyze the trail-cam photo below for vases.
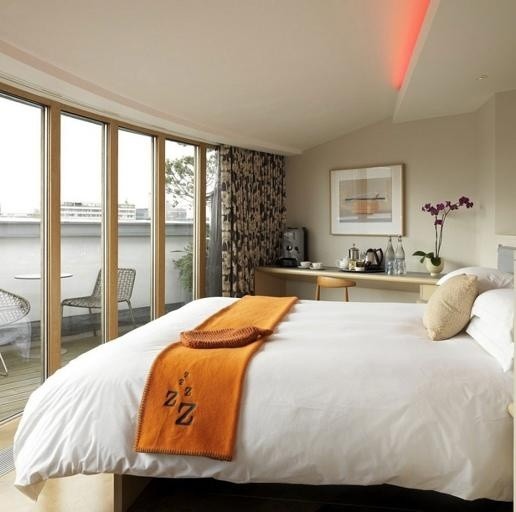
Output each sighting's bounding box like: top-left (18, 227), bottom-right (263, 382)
top-left (426, 257), bottom-right (444, 277)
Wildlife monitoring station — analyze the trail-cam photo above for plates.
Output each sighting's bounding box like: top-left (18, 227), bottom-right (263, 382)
top-left (309, 266), bottom-right (326, 270)
top-left (297, 266), bottom-right (311, 269)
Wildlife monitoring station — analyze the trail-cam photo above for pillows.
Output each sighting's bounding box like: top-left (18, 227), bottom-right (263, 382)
top-left (422, 264), bottom-right (514, 373)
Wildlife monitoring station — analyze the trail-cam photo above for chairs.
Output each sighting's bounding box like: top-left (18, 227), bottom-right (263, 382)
top-left (1, 289), bottom-right (31, 377)
top-left (61, 268), bottom-right (137, 337)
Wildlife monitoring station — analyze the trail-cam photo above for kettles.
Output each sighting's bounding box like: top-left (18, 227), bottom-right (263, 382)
top-left (364, 247), bottom-right (383, 272)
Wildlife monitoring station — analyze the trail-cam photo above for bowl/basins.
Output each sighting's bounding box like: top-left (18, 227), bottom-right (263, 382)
top-left (354, 267), bottom-right (365, 271)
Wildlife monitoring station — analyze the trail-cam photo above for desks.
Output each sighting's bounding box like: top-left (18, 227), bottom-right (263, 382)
top-left (254, 264), bottom-right (446, 301)
top-left (14, 273), bottom-right (73, 359)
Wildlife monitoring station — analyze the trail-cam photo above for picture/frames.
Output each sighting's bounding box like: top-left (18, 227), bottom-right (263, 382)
top-left (330, 163), bottom-right (404, 237)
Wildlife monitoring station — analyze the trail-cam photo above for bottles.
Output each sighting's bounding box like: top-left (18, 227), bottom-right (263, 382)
top-left (384, 235), bottom-right (395, 274)
top-left (349, 243), bottom-right (360, 260)
top-left (394, 235), bottom-right (406, 275)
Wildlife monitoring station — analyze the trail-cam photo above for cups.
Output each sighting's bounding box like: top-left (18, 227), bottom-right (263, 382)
top-left (300, 261), bottom-right (311, 267)
top-left (311, 262), bottom-right (324, 269)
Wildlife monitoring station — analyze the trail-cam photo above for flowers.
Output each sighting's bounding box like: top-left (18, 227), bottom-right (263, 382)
top-left (412, 196), bottom-right (473, 266)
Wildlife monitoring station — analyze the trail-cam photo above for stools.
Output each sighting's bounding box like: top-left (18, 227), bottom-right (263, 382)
top-left (315, 275), bottom-right (356, 302)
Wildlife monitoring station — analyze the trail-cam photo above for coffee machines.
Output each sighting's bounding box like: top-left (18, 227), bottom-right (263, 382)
top-left (278, 226), bottom-right (307, 267)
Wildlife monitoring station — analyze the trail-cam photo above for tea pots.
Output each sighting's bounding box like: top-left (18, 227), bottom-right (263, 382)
top-left (335, 255), bottom-right (350, 269)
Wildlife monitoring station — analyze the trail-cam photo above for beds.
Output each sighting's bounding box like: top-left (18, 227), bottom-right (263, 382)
top-left (13, 234), bottom-right (514, 512)
